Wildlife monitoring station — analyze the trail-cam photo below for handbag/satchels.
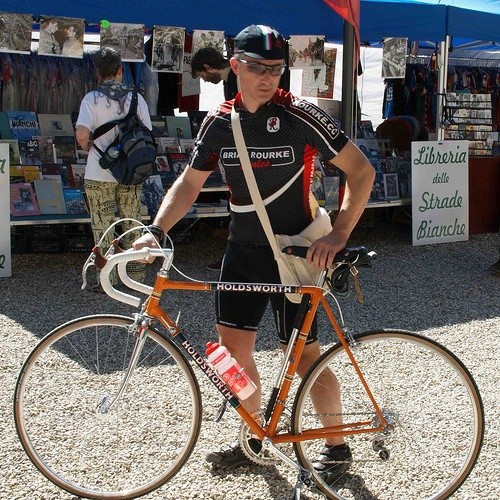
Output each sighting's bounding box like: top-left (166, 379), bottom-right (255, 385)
top-left (272, 208), bottom-right (336, 303)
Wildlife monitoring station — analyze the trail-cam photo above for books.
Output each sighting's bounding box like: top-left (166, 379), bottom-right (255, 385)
top-left (0, 110), bottom-right (196, 215)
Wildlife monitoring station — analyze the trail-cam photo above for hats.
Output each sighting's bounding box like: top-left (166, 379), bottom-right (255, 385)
top-left (234, 24), bottom-right (287, 60)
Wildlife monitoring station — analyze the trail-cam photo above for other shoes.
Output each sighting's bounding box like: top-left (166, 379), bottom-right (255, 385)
top-left (489, 259), bottom-right (500, 276)
top-left (88, 282), bottom-right (104, 293)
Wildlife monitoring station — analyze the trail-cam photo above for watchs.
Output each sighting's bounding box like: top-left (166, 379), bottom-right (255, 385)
top-left (142, 224), bottom-right (164, 242)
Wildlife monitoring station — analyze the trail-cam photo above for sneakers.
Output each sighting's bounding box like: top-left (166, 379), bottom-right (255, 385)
top-left (299, 445), bottom-right (352, 483)
top-left (205, 438), bottom-right (268, 470)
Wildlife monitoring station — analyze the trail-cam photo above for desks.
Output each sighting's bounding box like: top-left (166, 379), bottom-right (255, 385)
top-left (468, 155), bottom-right (500, 234)
top-left (10, 201), bottom-right (408, 236)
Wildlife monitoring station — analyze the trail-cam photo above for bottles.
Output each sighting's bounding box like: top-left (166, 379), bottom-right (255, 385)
top-left (99, 144), bottom-right (121, 169)
top-left (206, 342), bottom-right (258, 401)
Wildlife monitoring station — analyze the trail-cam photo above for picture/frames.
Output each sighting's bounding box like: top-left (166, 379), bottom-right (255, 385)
top-left (383, 174), bottom-right (400, 198)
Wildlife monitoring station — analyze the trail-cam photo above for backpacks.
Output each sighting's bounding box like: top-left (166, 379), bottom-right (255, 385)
top-left (93, 91), bottom-right (156, 186)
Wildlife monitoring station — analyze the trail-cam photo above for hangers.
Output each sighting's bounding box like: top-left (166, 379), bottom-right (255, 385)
top-left (406, 54), bottom-right (500, 67)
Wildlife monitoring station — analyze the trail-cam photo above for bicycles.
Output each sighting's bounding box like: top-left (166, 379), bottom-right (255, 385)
top-left (14, 218), bottom-right (485, 500)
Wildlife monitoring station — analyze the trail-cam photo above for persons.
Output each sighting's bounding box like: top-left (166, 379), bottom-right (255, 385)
top-left (75, 47), bottom-right (153, 293)
top-left (130, 25), bottom-right (377, 488)
top-left (190, 48), bottom-right (238, 272)
top-left (1, 12), bottom-right (221, 73)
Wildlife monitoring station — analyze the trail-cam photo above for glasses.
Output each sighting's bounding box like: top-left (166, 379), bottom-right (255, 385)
top-left (197, 70), bottom-right (203, 79)
top-left (237, 58), bottom-right (287, 77)
